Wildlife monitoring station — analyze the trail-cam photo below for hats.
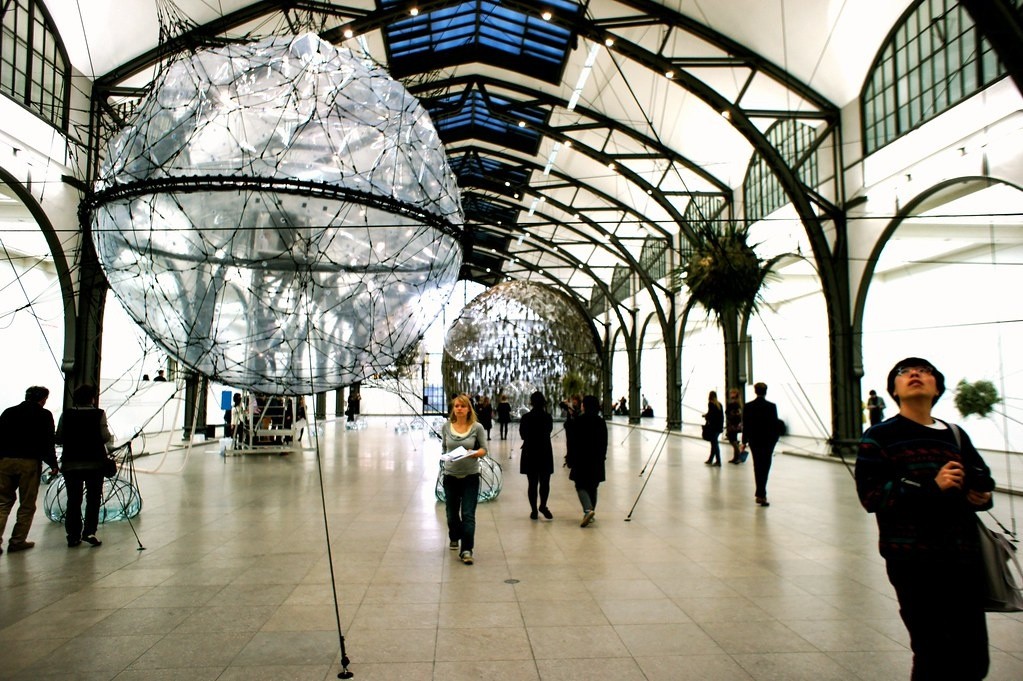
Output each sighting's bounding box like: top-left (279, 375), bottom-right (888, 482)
top-left (887, 357), bottom-right (946, 406)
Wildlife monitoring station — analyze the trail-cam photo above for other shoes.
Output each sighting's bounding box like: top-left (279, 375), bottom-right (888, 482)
top-left (539, 505), bottom-right (553, 519)
top-left (760, 502), bottom-right (770, 506)
top-left (81, 533), bottom-right (104, 546)
top-left (729, 455), bottom-right (742, 464)
top-left (487, 438), bottom-right (491, 440)
top-left (580, 510), bottom-right (594, 527)
top-left (713, 461), bottom-right (721, 466)
top-left (7, 540), bottom-right (34, 552)
top-left (755, 498), bottom-right (762, 504)
top-left (68, 540), bottom-right (81, 547)
top-left (705, 459), bottom-right (714, 463)
top-left (530, 510), bottom-right (538, 519)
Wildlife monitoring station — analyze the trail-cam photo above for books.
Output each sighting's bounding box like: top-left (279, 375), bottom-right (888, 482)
top-left (440, 444), bottom-right (478, 462)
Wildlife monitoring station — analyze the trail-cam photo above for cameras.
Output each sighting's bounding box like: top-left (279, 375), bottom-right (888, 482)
top-left (960, 465), bottom-right (995, 495)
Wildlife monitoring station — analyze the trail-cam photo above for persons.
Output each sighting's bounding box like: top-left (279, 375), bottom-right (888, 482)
top-left (561, 392), bottom-right (608, 528)
top-left (702, 391), bottom-right (724, 468)
top-left (154, 370), bottom-right (168, 382)
top-left (0, 386), bottom-right (59, 555)
top-left (448, 392), bottom-right (495, 441)
top-left (738, 383), bottom-right (781, 507)
top-left (441, 395), bottom-right (489, 564)
top-left (142, 374), bottom-right (149, 380)
top-left (725, 389), bottom-right (748, 465)
top-left (227, 392), bottom-right (308, 450)
top-left (867, 390), bottom-right (886, 427)
top-left (855, 358), bottom-right (996, 681)
top-left (642, 393), bottom-right (648, 409)
top-left (611, 396), bottom-right (628, 415)
top-left (496, 394), bottom-right (512, 440)
top-left (519, 391), bottom-right (556, 519)
top-left (54, 382), bottom-right (112, 549)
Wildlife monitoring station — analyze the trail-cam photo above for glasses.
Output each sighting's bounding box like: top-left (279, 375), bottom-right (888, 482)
top-left (897, 368), bottom-right (931, 378)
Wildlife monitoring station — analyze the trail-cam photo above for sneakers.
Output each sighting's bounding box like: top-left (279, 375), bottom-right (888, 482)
top-left (450, 541), bottom-right (459, 549)
top-left (460, 550), bottom-right (473, 564)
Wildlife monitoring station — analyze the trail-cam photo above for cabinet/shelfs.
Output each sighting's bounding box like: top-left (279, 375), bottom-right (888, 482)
top-left (221, 394), bottom-right (297, 454)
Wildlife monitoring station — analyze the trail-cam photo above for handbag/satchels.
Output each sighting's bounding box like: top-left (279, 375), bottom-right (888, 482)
top-left (345, 407), bottom-right (350, 415)
top-left (949, 423), bottom-right (1023, 613)
top-left (106, 458), bottom-right (117, 478)
top-left (702, 424), bottom-right (709, 441)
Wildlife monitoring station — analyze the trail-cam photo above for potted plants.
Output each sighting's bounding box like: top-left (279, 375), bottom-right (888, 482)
top-left (952, 378), bottom-right (1004, 420)
